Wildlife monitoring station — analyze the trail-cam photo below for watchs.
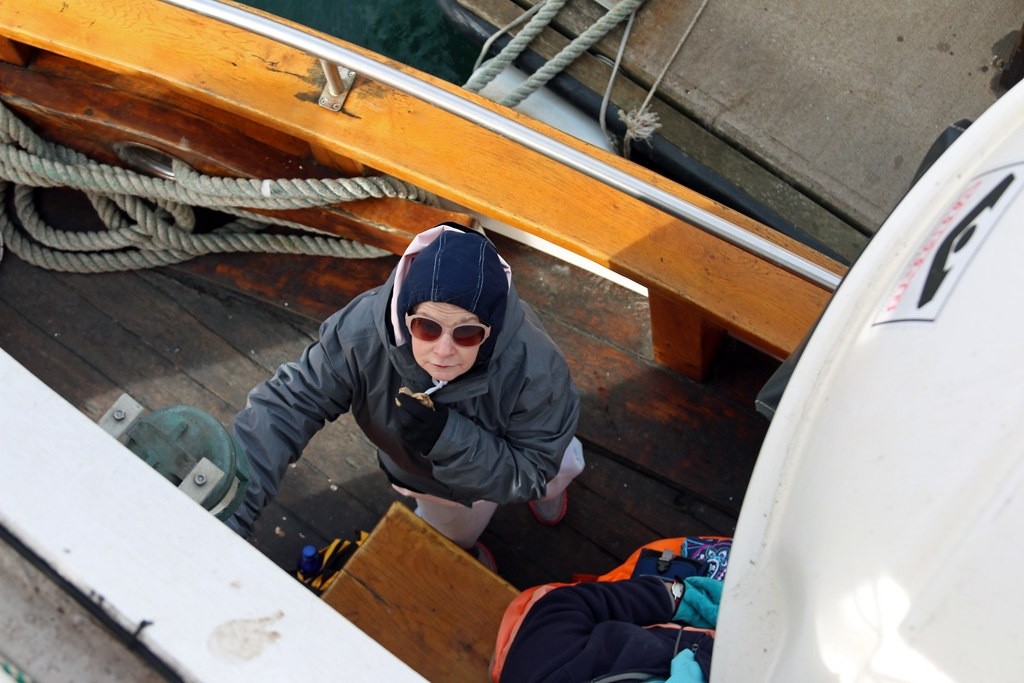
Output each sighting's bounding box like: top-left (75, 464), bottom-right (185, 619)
top-left (672, 581), bottom-right (684, 599)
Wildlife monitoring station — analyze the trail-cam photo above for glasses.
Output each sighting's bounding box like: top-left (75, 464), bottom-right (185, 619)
top-left (405, 312), bottom-right (491, 349)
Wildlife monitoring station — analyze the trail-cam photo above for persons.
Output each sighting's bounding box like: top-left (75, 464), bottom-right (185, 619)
top-left (224, 221), bottom-right (584, 575)
top-left (488, 537), bottom-right (733, 683)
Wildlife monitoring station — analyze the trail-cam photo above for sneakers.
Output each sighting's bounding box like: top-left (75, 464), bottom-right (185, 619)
top-left (470, 541), bottom-right (497, 574)
top-left (528, 489), bottom-right (567, 527)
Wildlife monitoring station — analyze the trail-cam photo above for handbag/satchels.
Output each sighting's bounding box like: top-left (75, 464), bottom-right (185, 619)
top-left (630, 547), bottom-right (709, 585)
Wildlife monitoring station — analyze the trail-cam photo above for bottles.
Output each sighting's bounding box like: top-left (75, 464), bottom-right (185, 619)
top-left (296, 545), bottom-right (322, 577)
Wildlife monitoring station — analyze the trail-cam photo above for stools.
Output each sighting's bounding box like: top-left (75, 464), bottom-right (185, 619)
top-left (322, 503), bottom-right (524, 683)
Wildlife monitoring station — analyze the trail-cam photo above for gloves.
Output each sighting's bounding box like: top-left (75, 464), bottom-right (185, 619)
top-left (395, 392), bottom-right (447, 456)
top-left (671, 576), bottom-right (725, 629)
